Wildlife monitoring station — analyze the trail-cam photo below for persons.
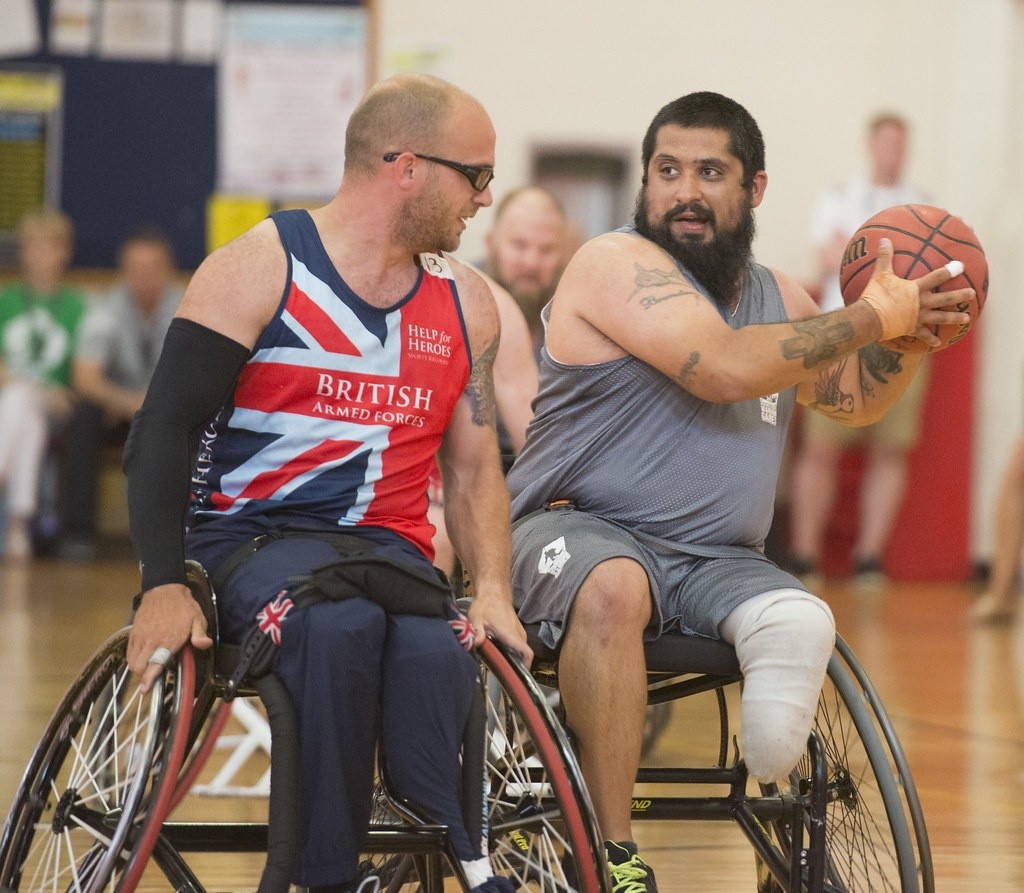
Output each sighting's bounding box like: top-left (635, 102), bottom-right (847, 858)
top-left (125, 73), bottom-right (532, 893)
top-left (1, 111), bottom-right (1024, 622)
top-left (505, 91), bottom-right (975, 893)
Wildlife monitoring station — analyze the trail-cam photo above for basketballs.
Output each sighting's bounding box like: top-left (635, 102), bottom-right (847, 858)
top-left (840, 204), bottom-right (991, 355)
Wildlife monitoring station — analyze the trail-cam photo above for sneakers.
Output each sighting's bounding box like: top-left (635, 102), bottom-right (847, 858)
top-left (592, 839), bottom-right (657, 893)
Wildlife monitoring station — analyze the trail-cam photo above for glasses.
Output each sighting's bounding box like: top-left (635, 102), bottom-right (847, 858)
top-left (383, 152), bottom-right (496, 192)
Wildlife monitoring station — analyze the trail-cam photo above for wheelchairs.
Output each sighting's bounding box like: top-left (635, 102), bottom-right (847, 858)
top-left (1, 558), bottom-right (934, 891)
